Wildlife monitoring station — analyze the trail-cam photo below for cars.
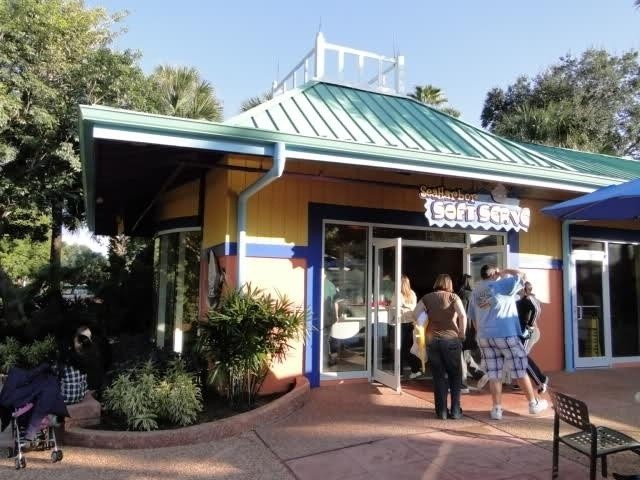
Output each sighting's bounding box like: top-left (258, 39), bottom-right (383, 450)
top-left (327, 259), bottom-right (394, 327)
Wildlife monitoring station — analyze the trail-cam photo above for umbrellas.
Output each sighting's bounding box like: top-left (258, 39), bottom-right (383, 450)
top-left (540, 178), bottom-right (640, 221)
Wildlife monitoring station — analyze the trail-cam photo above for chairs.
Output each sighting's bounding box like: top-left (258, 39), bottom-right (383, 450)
top-left (546, 388), bottom-right (640, 480)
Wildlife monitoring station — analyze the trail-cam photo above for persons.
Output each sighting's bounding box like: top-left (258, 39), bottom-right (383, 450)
top-left (59, 335), bottom-right (95, 405)
top-left (77, 325), bottom-right (104, 376)
top-left (323, 264), bottom-right (549, 420)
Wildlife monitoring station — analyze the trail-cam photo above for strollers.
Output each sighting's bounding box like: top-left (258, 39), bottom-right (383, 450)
top-left (3, 364), bottom-right (64, 470)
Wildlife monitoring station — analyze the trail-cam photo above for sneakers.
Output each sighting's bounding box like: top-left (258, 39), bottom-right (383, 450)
top-left (409, 371), bottom-right (470, 420)
top-left (528, 397), bottom-right (549, 416)
top-left (477, 374), bottom-right (491, 390)
top-left (536, 374), bottom-right (549, 395)
top-left (490, 405), bottom-right (502, 420)
